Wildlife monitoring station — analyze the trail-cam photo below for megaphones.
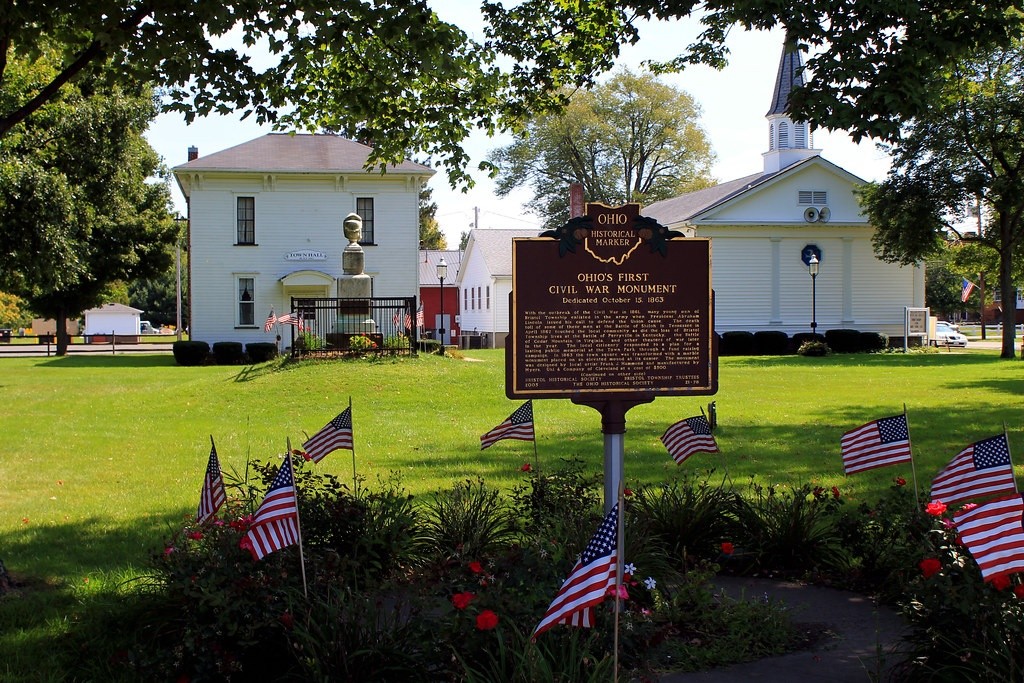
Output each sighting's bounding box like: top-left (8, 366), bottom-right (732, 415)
top-left (804, 207), bottom-right (819, 223)
top-left (819, 207), bottom-right (831, 223)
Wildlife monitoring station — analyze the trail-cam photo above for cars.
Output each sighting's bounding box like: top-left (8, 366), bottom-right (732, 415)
top-left (924, 324), bottom-right (968, 347)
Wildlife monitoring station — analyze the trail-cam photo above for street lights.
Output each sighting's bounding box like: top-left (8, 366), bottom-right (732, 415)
top-left (808, 254), bottom-right (819, 340)
top-left (436, 256), bottom-right (448, 345)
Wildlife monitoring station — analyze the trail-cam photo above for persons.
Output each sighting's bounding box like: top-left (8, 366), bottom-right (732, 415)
top-left (19, 326), bottom-right (25, 336)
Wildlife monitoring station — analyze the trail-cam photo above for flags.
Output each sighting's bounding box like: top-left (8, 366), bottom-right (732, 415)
top-left (839, 413), bottom-right (911, 474)
top-left (480, 400), bottom-right (535, 450)
top-left (532, 503), bottom-right (617, 642)
top-left (952, 493), bottom-right (1024, 582)
top-left (279, 313), bottom-right (298, 326)
top-left (265, 308), bottom-right (277, 332)
top-left (302, 407), bottom-right (353, 464)
top-left (299, 314), bottom-right (304, 332)
top-left (394, 314), bottom-right (399, 325)
top-left (246, 456), bottom-right (300, 561)
top-left (962, 280), bottom-right (974, 302)
top-left (660, 415), bottom-right (718, 466)
top-left (405, 304), bottom-right (423, 330)
top-left (195, 446), bottom-right (226, 527)
top-left (930, 431), bottom-right (1016, 504)
